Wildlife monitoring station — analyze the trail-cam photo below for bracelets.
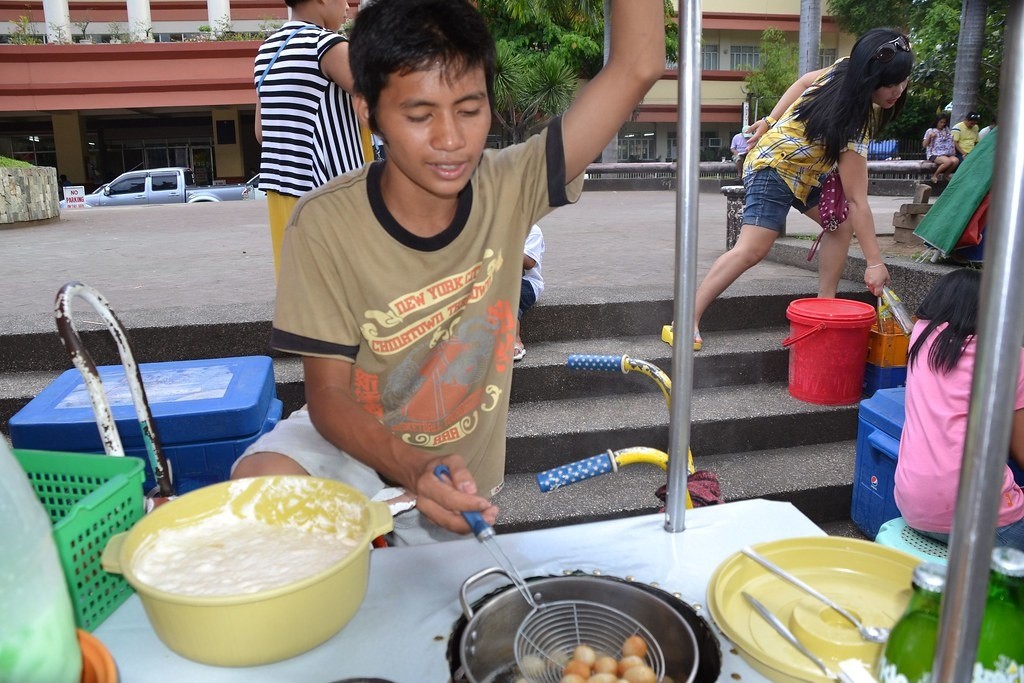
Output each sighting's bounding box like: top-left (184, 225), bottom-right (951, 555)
top-left (763, 117), bottom-right (771, 131)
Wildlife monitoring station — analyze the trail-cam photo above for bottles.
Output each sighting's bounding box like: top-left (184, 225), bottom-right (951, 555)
top-left (877, 294), bottom-right (896, 334)
top-left (878, 561), bottom-right (946, 683)
top-left (969, 545), bottom-right (1024, 683)
top-left (880, 284), bottom-right (915, 339)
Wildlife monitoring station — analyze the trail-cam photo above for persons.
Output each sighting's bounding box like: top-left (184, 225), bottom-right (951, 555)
top-left (893, 263), bottom-right (1024, 556)
top-left (661, 27), bottom-right (912, 350)
top-left (254, 0), bottom-right (546, 361)
top-left (731, 110), bottom-right (996, 183)
top-left (229, 0), bottom-right (664, 550)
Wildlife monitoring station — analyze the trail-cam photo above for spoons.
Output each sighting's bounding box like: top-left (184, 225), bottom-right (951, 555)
top-left (740, 546), bottom-right (892, 644)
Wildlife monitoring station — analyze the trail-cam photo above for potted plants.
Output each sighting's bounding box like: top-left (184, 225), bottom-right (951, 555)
top-left (199, 25), bottom-right (212, 40)
top-left (257, 13), bottom-right (280, 38)
top-left (105, 19), bottom-right (122, 44)
top-left (64, 12), bottom-right (92, 44)
top-left (132, 17), bottom-right (156, 43)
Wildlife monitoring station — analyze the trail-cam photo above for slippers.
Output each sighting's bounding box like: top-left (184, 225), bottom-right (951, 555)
top-left (512, 346), bottom-right (526, 360)
top-left (661, 319), bottom-right (703, 351)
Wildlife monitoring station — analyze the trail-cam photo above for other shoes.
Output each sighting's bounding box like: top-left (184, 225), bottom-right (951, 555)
top-left (734, 176), bottom-right (742, 181)
top-left (945, 175), bottom-right (951, 182)
top-left (931, 176), bottom-right (937, 183)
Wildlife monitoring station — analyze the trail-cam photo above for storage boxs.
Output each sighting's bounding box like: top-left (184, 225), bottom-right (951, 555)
top-left (10, 356), bottom-right (285, 483)
top-left (9, 449), bottom-right (146, 634)
top-left (849, 389), bottom-right (910, 535)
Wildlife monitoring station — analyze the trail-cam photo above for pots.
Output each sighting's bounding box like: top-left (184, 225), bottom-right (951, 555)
top-left (458, 567), bottom-right (699, 683)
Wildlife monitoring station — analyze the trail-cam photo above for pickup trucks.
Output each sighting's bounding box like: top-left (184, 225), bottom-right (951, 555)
top-left (61, 166), bottom-right (255, 209)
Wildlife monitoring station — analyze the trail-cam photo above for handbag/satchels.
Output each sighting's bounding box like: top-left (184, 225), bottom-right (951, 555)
top-left (931, 134), bottom-right (956, 156)
top-left (807, 171), bottom-right (848, 261)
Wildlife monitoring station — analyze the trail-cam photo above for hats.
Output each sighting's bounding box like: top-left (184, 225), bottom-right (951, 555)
top-left (742, 125), bottom-right (754, 138)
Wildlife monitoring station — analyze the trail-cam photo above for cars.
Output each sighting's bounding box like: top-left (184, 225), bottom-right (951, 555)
top-left (240, 172), bottom-right (266, 198)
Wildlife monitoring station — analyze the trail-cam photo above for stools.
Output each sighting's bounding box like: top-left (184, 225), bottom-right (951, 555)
top-left (880, 517), bottom-right (952, 575)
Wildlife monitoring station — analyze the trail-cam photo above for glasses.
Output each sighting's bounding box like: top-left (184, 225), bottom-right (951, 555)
top-left (970, 114), bottom-right (981, 118)
top-left (866, 36), bottom-right (910, 68)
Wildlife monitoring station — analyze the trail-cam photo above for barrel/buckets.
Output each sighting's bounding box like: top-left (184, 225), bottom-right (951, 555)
top-left (782, 298), bottom-right (877, 407)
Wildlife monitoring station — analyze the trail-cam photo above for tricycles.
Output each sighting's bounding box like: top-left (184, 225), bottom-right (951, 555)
top-left (56, 281), bottom-right (698, 541)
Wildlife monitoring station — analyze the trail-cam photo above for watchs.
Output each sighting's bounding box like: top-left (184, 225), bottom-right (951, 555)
top-left (766, 116), bottom-right (778, 127)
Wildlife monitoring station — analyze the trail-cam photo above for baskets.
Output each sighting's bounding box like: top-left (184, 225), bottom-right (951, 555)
top-left (8, 449), bottom-right (146, 634)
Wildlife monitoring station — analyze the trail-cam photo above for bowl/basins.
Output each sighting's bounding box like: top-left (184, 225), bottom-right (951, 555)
top-left (706, 536), bottom-right (925, 682)
top-left (100, 474), bottom-right (395, 667)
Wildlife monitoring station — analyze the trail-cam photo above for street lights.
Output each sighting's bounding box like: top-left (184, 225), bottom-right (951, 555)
top-left (28, 135), bottom-right (40, 164)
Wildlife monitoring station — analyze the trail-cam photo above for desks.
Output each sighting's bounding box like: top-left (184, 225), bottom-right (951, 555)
top-left (0, 498), bottom-right (841, 682)
